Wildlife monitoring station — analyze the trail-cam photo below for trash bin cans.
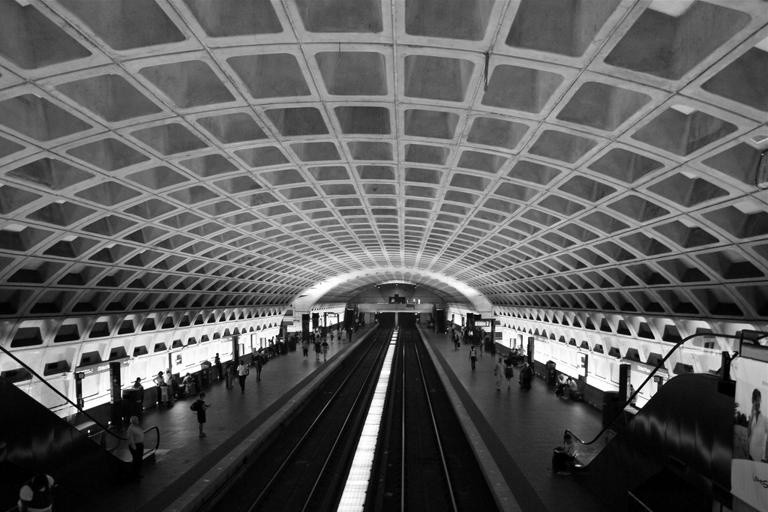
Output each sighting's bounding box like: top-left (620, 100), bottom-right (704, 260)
top-left (123, 390), bottom-right (140, 426)
top-left (602, 391), bottom-right (620, 428)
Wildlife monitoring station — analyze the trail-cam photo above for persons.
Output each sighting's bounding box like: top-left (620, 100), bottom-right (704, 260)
top-left (257, 331), bottom-right (300, 364)
top-left (521, 359), bottom-right (534, 392)
top-left (555, 374), bottom-right (569, 396)
top-left (630, 384), bottom-right (637, 405)
top-left (134, 376), bottom-right (145, 410)
top-left (255, 354), bottom-right (262, 383)
top-left (166, 370), bottom-right (201, 409)
top-left (561, 376), bottom-right (578, 399)
top-left (469, 345), bottom-right (477, 370)
top-left (237, 360), bottom-right (247, 393)
top-left (17, 472), bottom-right (56, 512)
top-left (194, 392), bottom-right (212, 438)
top-left (152, 370), bottom-right (167, 407)
top-left (215, 353), bottom-right (224, 380)
top-left (745, 387), bottom-right (767, 461)
top-left (295, 314), bottom-right (379, 362)
top-left (128, 415), bottom-right (145, 478)
top-left (504, 361), bottom-right (513, 384)
top-left (495, 358), bottom-right (504, 391)
top-left (223, 359), bottom-right (238, 391)
top-left (425, 316), bottom-right (496, 358)
top-left (504, 344), bottom-right (526, 368)
